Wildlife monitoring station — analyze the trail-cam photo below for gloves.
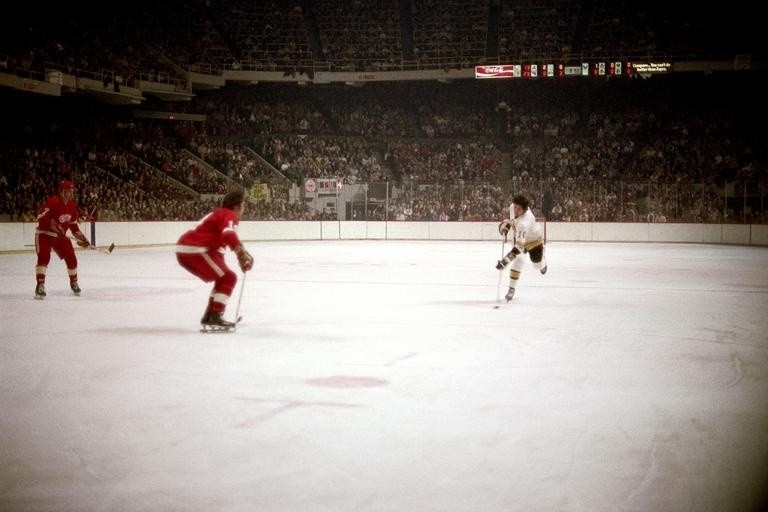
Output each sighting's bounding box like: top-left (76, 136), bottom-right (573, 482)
top-left (237, 248), bottom-right (253, 272)
top-left (499, 220), bottom-right (512, 236)
top-left (77, 235), bottom-right (90, 248)
top-left (496, 258), bottom-right (508, 270)
top-left (53, 223), bottom-right (63, 235)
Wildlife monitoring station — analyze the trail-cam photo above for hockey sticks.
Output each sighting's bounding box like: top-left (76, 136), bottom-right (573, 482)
top-left (494, 233), bottom-right (506, 308)
top-left (233, 269), bottom-right (246, 325)
top-left (70, 237), bottom-right (114, 255)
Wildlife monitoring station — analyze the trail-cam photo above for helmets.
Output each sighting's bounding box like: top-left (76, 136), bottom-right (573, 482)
top-left (58, 180), bottom-right (75, 192)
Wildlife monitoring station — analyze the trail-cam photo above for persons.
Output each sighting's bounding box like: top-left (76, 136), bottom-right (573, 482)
top-left (31, 180), bottom-right (90, 296)
top-left (1, 0), bottom-right (768, 224)
top-left (494, 194), bottom-right (547, 302)
top-left (174, 190), bottom-right (255, 328)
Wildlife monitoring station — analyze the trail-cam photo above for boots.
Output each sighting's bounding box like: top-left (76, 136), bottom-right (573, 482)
top-left (70, 282), bottom-right (81, 293)
top-left (35, 283), bottom-right (46, 296)
top-left (539, 260), bottom-right (548, 275)
top-left (207, 313), bottom-right (237, 327)
top-left (200, 307), bottom-right (211, 325)
top-left (505, 286), bottom-right (516, 301)
